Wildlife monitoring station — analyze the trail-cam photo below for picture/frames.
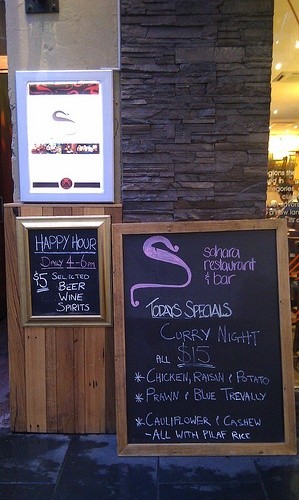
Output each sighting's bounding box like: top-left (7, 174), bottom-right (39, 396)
top-left (16, 215), bottom-right (111, 327)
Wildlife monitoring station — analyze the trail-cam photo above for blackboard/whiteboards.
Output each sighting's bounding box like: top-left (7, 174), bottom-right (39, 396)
top-left (14, 215), bottom-right (113, 328)
top-left (110, 218), bottom-right (298, 458)
